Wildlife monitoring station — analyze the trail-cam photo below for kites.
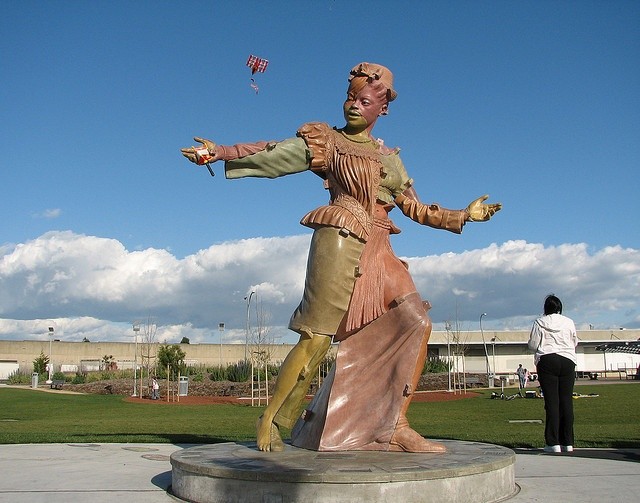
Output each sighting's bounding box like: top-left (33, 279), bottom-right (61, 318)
top-left (246, 53), bottom-right (268, 94)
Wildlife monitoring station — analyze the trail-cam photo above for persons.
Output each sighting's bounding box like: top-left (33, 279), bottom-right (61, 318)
top-left (180, 61), bottom-right (503, 456)
top-left (527, 294), bottom-right (579, 453)
top-left (517, 362), bottom-right (530, 389)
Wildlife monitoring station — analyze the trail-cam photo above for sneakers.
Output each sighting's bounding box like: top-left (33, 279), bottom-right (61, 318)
top-left (562, 445), bottom-right (573, 452)
top-left (544, 444), bottom-right (561, 453)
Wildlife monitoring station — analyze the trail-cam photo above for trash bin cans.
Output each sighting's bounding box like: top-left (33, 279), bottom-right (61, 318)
top-left (179, 375), bottom-right (188, 397)
top-left (32, 372), bottom-right (38, 389)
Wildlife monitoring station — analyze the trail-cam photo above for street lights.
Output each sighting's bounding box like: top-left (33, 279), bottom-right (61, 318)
top-left (133, 321), bottom-right (140, 394)
top-left (219, 322), bottom-right (225, 364)
top-left (480, 312), bottom-right (491, 370)
top-left (445, 322), bottom-right (453, 389)
top-left (244, 291), bottom-right (255, 364)
top-left (47, 326), bottom-right (54, 383)
